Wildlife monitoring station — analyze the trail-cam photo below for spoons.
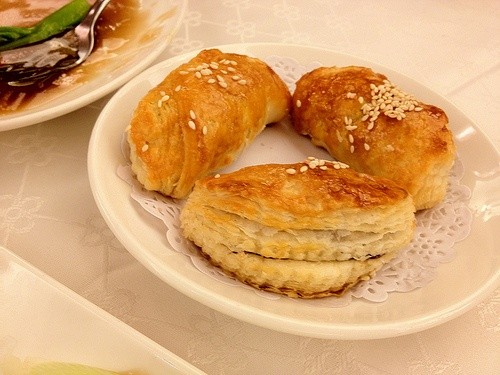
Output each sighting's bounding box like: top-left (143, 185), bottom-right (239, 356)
top-left (50, 0), bottom-right (110, 70)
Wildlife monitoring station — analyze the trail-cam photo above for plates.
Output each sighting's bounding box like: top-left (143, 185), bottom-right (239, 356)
top-left (88, 43), bottom-right (500, 340)
top-left (0, 244), bottom-right (208, 375)
top-left (0, 0), bottom-right (188, 131)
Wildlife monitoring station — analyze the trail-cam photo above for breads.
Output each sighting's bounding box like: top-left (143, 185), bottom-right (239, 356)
top-left (180, 161), bottom-right (417, 294)
top-left (291, 67), bottom-right (456, 212)
top-left (126, 48), bottom-right (291, 199)
